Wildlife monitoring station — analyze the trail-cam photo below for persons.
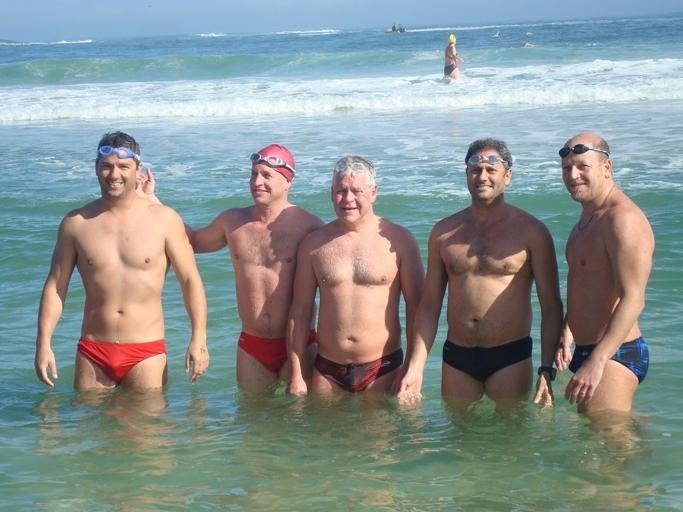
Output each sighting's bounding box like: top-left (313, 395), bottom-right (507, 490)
top-left (443, 34), bottom-right (465, 82)
top-left (33, 131), bottom-right (211, 390)
top-left (554, 130), bottom-right (656, 414)
top-left (399, 135), bottom-right (565, 411)
top-left (284, 155), bottom-right (426, 397)
top-left (134, 143), bottom-right (328, 382)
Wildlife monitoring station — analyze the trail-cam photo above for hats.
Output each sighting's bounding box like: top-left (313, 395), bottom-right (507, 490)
top-left (253, 144), bottom-right (295, 181)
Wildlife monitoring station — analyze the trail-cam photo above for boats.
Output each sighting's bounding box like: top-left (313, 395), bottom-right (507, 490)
top-left (384, 26), bottom-right (405, 33)
top-left (525, 30), bottom-right (535, 36)
top-left (520, 40), bottom-right (536, 49)
top-left (490, 31), bottom-right (503, 39)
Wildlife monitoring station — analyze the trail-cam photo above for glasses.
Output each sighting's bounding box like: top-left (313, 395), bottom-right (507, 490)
top-left (249, 152), bottom-right (296, 182)
top-left (559, 144), bottom-right (610, 159)
top-left (466, 155), bottom-right (509, 168)
top-left (97, 145), bottom-right (141, 163)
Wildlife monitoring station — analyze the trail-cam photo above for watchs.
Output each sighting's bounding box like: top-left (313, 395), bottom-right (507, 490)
top-left (537, 365), bottom-right (559, 383)
